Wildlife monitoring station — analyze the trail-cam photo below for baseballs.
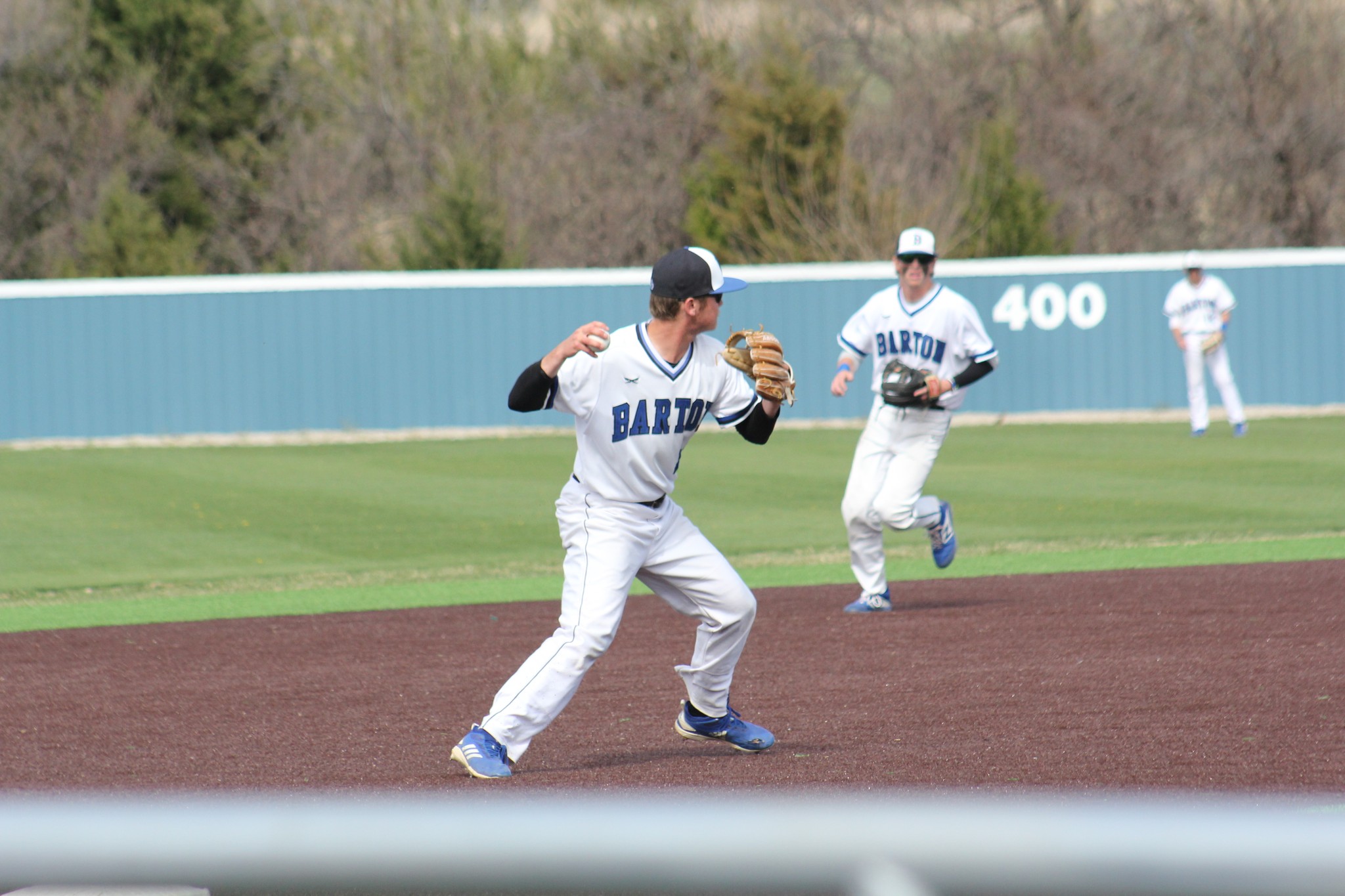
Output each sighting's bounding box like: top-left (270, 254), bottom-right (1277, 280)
top-left (586, 328), bottom-right (612, 353)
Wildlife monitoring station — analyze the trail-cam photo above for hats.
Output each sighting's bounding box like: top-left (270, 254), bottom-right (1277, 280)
top-left (649, 246), bottom-right (748, 298)
top-left (1183, 253), bottom-right (1204, 268)
top-left (897, 227), bottom-right (936, 257)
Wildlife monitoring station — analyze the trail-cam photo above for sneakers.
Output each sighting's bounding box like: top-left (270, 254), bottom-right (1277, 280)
top-left (1232, 422), bottom-right (1248, 436)
top-left (928, 500), bottom-right (957, 568)
top-left (1190, 428), bottom-right (1208, 437)
top-left (673, 699), bottom-right (775, 753)
top-left (450, 723), bottom-right (512, 779)
top-left (843, 587), bottom-right (892, 613)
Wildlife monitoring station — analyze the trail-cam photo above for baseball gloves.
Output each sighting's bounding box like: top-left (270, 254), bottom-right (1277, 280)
top-left (714, 321), bottom-right (799, 408)
top-left (880, 357), bottom-right (946, 412)
top-left (1200, 330), bottom-right (1225, 358)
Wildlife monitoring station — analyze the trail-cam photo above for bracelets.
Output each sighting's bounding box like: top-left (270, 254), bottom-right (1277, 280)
top-left (838, 364), bottom-right (850, 371)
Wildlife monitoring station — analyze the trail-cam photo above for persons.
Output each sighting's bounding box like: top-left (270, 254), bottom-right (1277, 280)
top-left (829, 227), bottom-right (1000, 612)
top-left (451, 243), bottom-right (796, 779)
top-left (1163, 248), bottom-right (1250, 439)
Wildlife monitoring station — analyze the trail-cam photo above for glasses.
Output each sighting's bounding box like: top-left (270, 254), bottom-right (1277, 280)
top-left (677, 294), bottom-right (724, 303)
top-left (900, 254), bottom-right (934, 263)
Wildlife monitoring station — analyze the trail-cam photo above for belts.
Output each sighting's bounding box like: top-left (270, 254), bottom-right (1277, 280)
top-left (885, 396), bottom-right (944, 411)
top-left (573, 473), bottom-right (666, 509)
